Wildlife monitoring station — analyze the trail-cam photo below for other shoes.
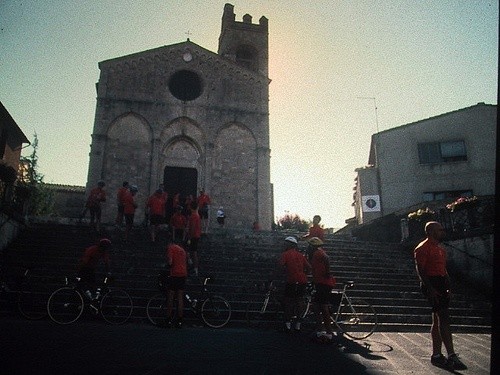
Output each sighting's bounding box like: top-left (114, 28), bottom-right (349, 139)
top-left (448, 353), bottom-right (468, 370)
top-left (431, 353), bottom-right (448, 365)
top-left (174, 318), bottom-right (184, 328)
top-left (159, 317), bottom-right (171, 329)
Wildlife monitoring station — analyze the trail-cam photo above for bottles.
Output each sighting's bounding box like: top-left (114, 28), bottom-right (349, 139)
top-left (85, 290), bottom-right (94, 302)
top-left (184, 293), bottom-right (192, 302)
top-left (94, 288), bottom-right (101, 300)
top-left (192, 297), bottom-right (199, 308)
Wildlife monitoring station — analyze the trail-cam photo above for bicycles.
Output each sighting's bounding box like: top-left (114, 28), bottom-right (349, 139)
top-left (246, 281), bottom-right (320, 330)
top-left (145, 272), bottom-right (233, 328)
top-left (289, 281), bottom-right (378, 339)
top-left (46, 270), bottom-right (134, 325)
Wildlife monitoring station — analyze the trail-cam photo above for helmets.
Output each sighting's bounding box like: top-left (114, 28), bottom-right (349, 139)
top-left (98, 238), bottom-right (111, 248)
top-left (307, 237), bottom-right (324, 247)
top-left (285, 236), bottom-right (298, 245)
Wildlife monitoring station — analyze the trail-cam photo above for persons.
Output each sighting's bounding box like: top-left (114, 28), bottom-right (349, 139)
top-left (308, 237), bottom-right (336, 343)
top-left (217, 206), bottom-right (226, 229)
top-left (158, 239), bottom-right (188, 328)
top-left (123, 185), bottom-right (138, 243)
top-left (271, 236), bottom-right (308, 334)
top-left (86, 180), bottom-right (107, 233)
top-left (79, 239), bottom-right (111, 320)
top-left (114, 181), bottom-right (129, 227)
top-left (297, 216), bottom-right (324, 242)
top-left (414, 221), bottom-right (467, 371)
top-left (143, 184), bottom-right (212, 280)
